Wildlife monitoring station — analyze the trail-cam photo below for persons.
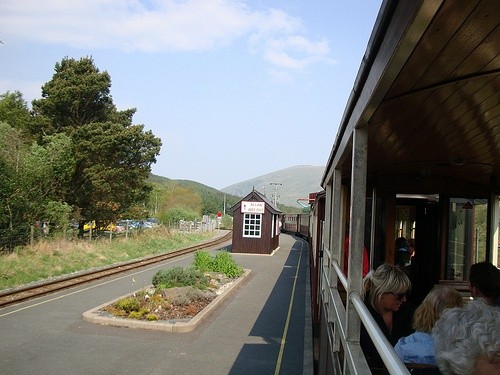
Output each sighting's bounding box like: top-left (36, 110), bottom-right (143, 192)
top-left (360, 264), bottom-right (411, 375)
top-left (395, 237), bottom-right (416, 271)
top-left (392, 261), bottom-right (500, 374)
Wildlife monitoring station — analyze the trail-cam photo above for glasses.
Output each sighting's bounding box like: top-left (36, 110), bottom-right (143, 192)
top-left (387, 289), bottom-right (409, 301)
